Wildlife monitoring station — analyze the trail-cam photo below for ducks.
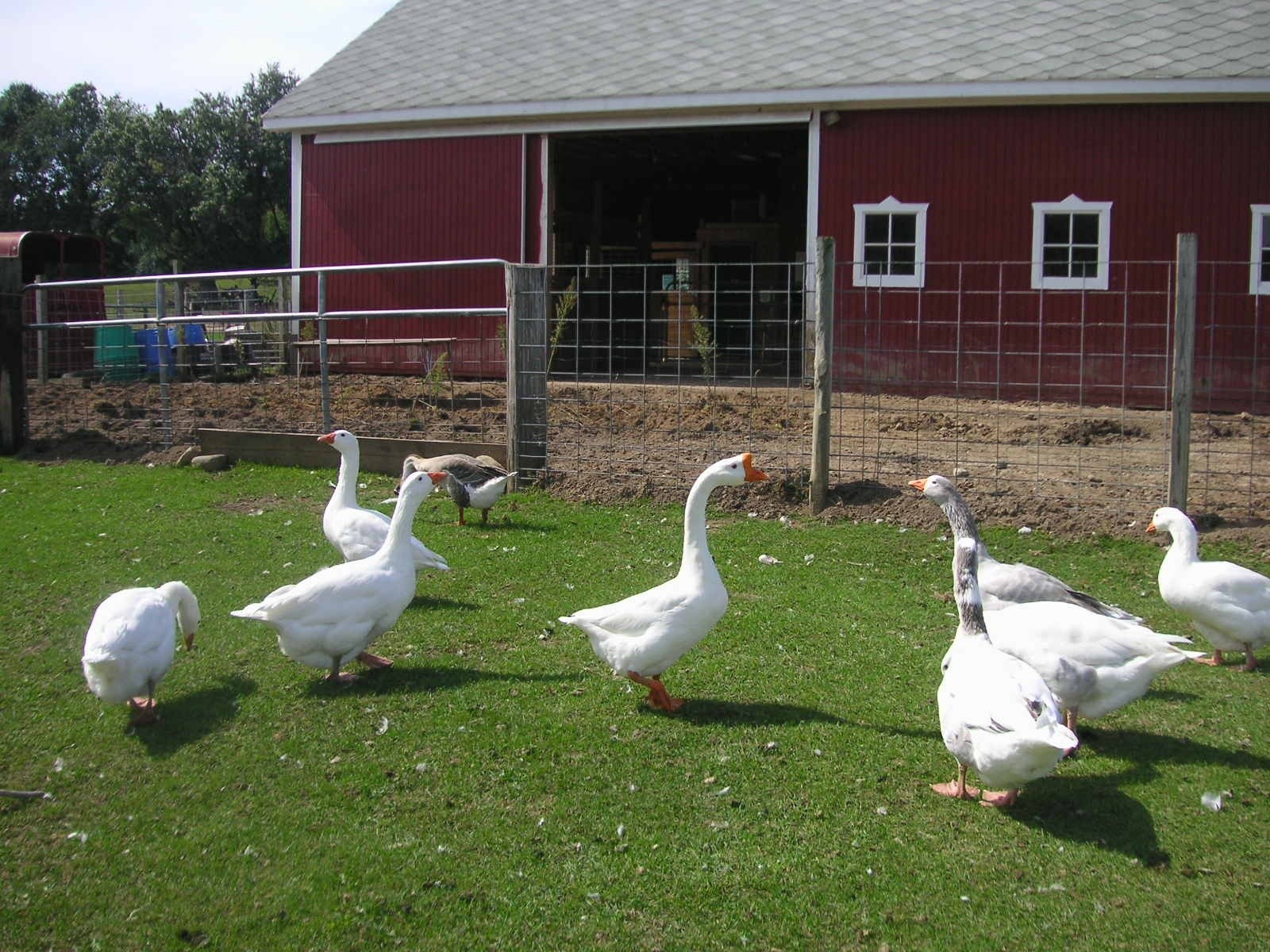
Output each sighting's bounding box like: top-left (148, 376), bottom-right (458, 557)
top-left (79, 580), bottom-right (201, 728)
top-left (1146, 507), bottom-right (1270, 671)
top-left (231, 430), bottom-right (519, 692)
top-left (908, 475), bottom-right (1207, 806)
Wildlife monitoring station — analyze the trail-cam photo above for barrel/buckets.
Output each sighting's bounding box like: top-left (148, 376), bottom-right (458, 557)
top-left (91, 327), bottom-right (140, 382)
top-left (166, 322), bottom-right (208, 347)
top-left (133, 328), bottom-right (175, 383)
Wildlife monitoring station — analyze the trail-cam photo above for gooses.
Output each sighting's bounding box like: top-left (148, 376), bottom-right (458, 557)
top-left (558, 453), bottom-right (770, 708)
